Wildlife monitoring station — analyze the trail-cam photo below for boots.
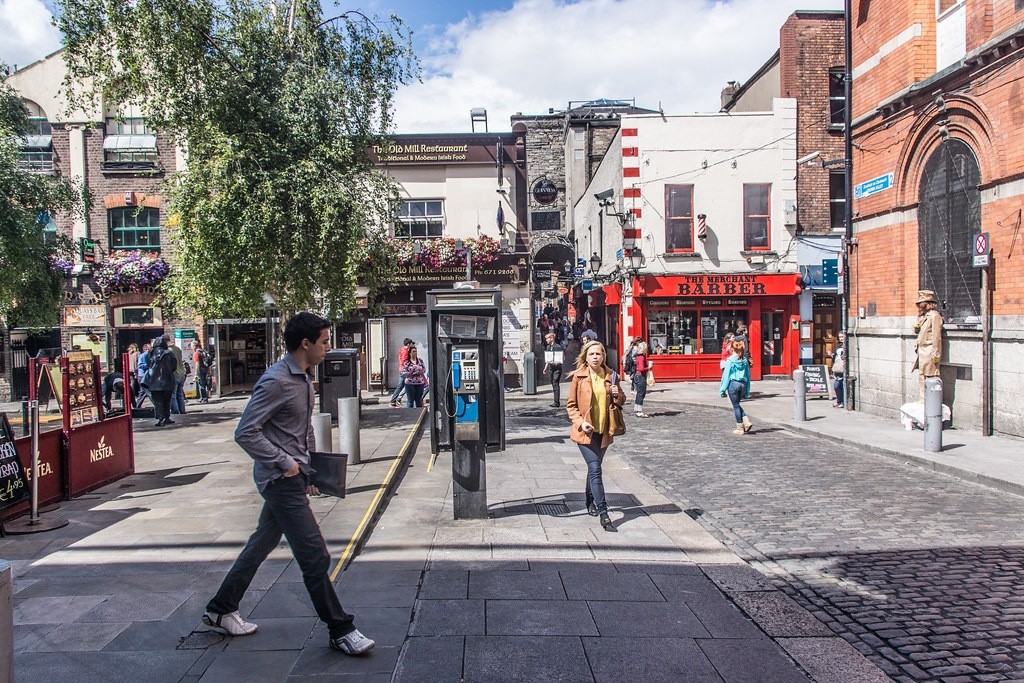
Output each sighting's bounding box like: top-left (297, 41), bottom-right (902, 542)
top-left (742, 415), bottom-right (753, 432)
top-left (733, 423), bottom-right (745, 435)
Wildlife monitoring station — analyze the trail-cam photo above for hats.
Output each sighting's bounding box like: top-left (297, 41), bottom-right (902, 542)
top-left (160, 333), bottom-right (171, 340)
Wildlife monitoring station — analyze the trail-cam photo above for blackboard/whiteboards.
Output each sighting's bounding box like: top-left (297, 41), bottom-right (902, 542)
top-left (802, 364), bottom-right (830, 394)
top-left (0, 411), bottom-right (33, 521)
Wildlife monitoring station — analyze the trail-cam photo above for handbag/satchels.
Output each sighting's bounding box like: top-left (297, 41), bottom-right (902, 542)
top-left (421, 373), bottom-right (428, 388)
top-left (308, 450), bottom-right (348, 499)
top-left (645, 367), bottom-right (655, 387)
top-left (608, 370), bottom-right (626, 437)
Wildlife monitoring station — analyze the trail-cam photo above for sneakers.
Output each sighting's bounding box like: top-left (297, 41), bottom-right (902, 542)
top-left (329, 628), bottom-right (376, 656)
top-left (202, 608), bottom-right (258, 636)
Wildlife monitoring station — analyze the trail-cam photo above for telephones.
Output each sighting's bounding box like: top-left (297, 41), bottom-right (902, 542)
top-left (453, 346), bottom-right (480, 393)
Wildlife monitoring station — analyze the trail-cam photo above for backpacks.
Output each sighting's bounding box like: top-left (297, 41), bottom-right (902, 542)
top-left (196, 348), bottom-right (212, 368)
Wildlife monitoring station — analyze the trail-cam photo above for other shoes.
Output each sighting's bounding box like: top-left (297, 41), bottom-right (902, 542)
top-left (833, 403), bottom-right (839, 407)
top-left (155, 418), bottom-right (166, 427)
top-left (600, 517), bottom-right (615, 531)
top-left (549, 403), bottom-right (559, 407)
top-left (165, 418), bottom-right (175, 424)
top-left (636, 411), bottom-right (649, 418)
top-left (585, 502), bottom-right (598, 516)
top-left (389, 401), bottom-right (403, 408)
top-left (838, 404), bottom-right (844, 408)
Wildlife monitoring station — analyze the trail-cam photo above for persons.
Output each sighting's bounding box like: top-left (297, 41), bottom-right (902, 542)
top-left (718, 319), bottom-right (754, 434)
top-left (827, 330), bottom-right (844, 408)
top-left (653, 339), bottom-right (662, 348)
top-left (390, 338), bottom-right (427, 408)
top-left (624, 337), bottom-right (653, 417)
top-left (148, 337), bottom-right (177, 426)
top-left (136, 344), bottom-right (154, 409)
top-left (191, 341), bottom-right (208, 403)
top-left (128, 343), bottom-right (141, 391)
top-left (913, 290), bottom-right (943, 401)
top-left (543, 333), bottom-right (565, 407)
top-left (537, 304), bottom-right (597, 350)
top-left (103, 372), bottom-right (136, 410)
top-left (161, 334), bottom-right (186, 414)
top-left (565, 341), bottom-right (627, 532)
top-left (203, 312), bottom-right (374, 655)
